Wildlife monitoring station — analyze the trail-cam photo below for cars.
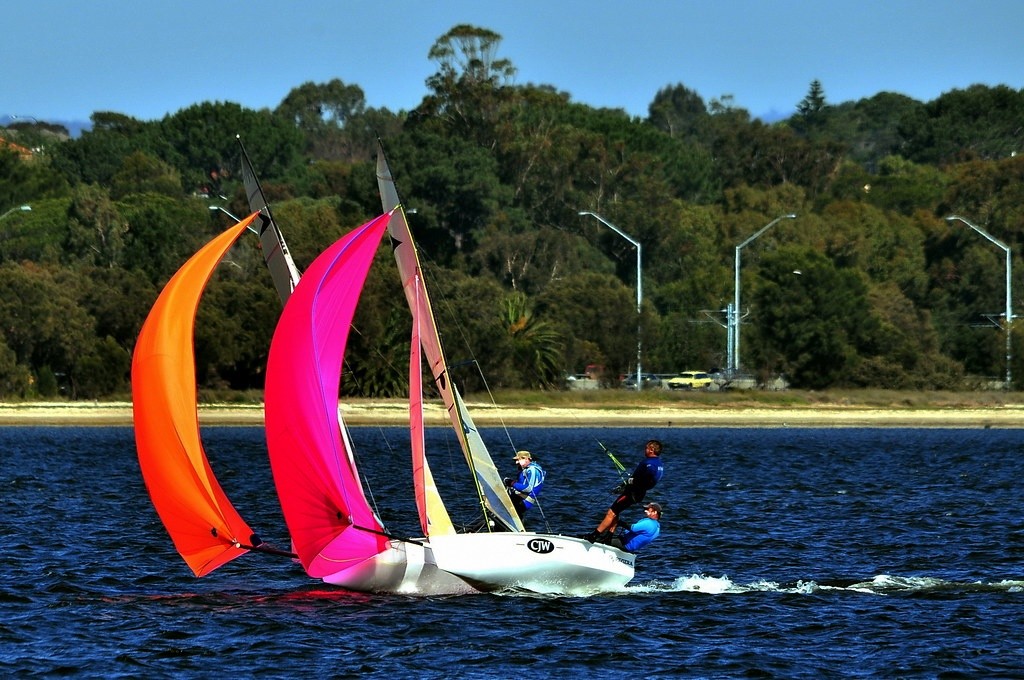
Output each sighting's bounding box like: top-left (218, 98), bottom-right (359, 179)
top-left (622, 373), bottom-right (662, 391)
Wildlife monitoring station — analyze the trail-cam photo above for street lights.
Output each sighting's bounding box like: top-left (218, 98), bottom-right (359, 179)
top-left (946, 216), bottom-right (1012, 388)
top-left (578, 210), bottom-right (642, 391)
top-left (735, 214), bottom-right (796, 370)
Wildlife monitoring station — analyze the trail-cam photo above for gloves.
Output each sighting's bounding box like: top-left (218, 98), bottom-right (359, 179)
top-left (505, 478), bottom-right (513, 487)
top-left (611, 486), bottom-right (623, 493)
top-left (626, 478), bottom-right (635, 485)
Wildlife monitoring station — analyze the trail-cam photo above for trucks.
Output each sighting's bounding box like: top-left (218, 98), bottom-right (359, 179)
top-left (586, 364), bottom-right (619, 389)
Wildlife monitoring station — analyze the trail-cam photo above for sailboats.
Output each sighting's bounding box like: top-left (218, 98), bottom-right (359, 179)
top-left (130, 131), bottom-right (637, 598)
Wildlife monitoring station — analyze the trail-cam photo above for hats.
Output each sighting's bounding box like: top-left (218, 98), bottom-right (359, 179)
top-left (643, 503), bottom-right (661, 514)
top-left (513, 451), bottom-right (531, 459)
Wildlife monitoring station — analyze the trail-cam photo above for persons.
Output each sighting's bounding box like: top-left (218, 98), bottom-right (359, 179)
top-left (617, 502), bottom-right (663, 553)
top-left (492, 450), bottom-right (546, 532)
top-left (577, 439), bottom-right (663, 546)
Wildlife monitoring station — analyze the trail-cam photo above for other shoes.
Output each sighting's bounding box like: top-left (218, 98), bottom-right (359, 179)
top-left (596, 535), bottom-right (612, 546)
top-left (577, 532), bottom-right (595, 544)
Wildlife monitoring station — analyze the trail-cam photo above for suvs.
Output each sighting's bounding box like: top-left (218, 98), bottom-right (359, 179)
top-left (668, 371), bottom-right (712, 390)
